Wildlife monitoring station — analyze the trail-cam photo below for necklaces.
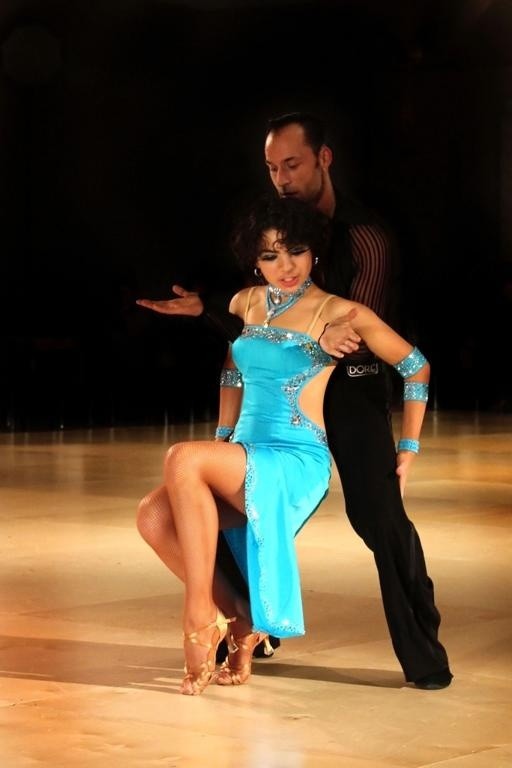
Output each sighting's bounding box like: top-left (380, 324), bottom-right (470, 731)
top-left (264, 278), bottom-right (312, 329)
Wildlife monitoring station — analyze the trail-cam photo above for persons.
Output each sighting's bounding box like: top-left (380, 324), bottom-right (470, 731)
top-left (134, 111), bottom-right (454, 689)
top-left (137, 199), bottom-right (431, 697)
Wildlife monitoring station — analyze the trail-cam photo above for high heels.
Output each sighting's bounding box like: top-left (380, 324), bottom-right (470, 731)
top-left (181, 604), bottom-right (280, 695)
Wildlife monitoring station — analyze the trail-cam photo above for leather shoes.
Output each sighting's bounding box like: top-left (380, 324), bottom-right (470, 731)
top-left (416, 670), bottom-right (452, 689)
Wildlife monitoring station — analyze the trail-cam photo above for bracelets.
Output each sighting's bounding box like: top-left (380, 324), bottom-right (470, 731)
top-left (403, 382), bottom-right (429, 402)
top-left (392, 346), bottom-right (428, 379)
top-left (215, 426), bottom-right (234, 438)
top-left (220, 368), bottom-right (244, 388)
top-left (397, 438), bottom-right (419, 454)
